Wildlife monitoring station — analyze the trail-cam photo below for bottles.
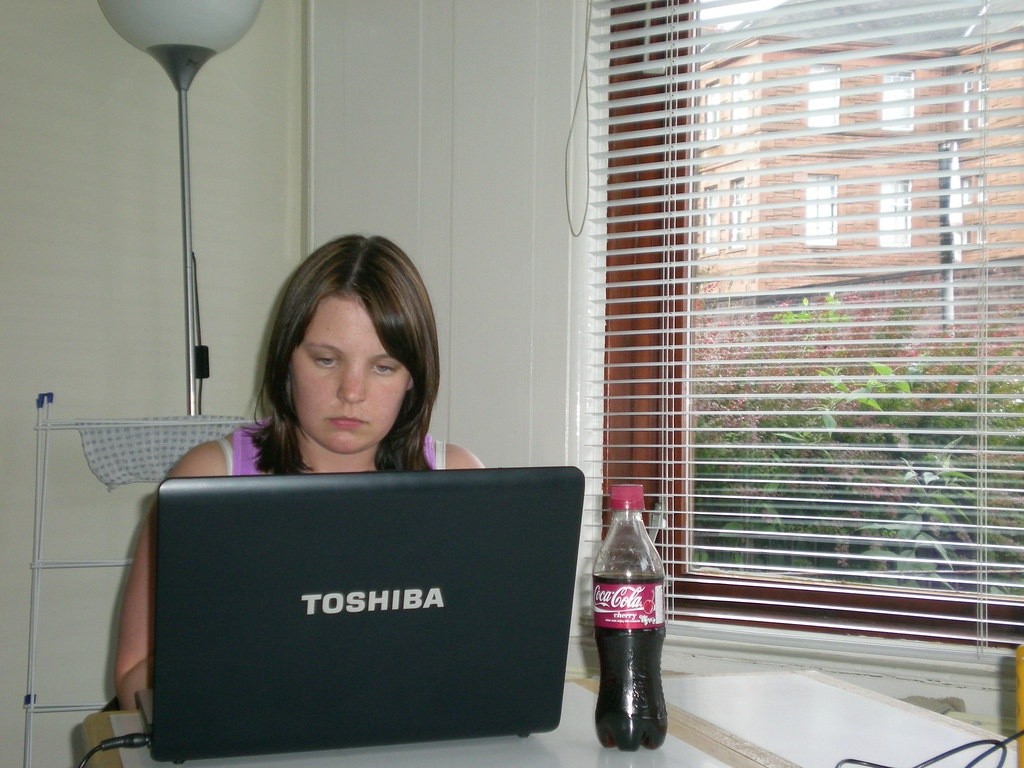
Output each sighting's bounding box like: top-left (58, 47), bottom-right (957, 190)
top-left (592, 484), bottom-right (668, 754)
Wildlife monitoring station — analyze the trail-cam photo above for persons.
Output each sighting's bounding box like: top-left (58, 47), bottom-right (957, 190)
top-left (116, 233), bottom-right (485, 721)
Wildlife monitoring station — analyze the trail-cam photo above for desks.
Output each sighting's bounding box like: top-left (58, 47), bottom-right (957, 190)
top-left (82, 669), bottom-right (1017, 768)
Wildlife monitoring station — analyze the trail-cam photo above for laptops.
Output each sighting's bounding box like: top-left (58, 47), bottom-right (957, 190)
top-left (136, 466), bottom-right (585, 766)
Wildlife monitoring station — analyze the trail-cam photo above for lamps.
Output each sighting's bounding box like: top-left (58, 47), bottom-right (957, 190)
top-left (98, 0), bottom-right (264, 416)
